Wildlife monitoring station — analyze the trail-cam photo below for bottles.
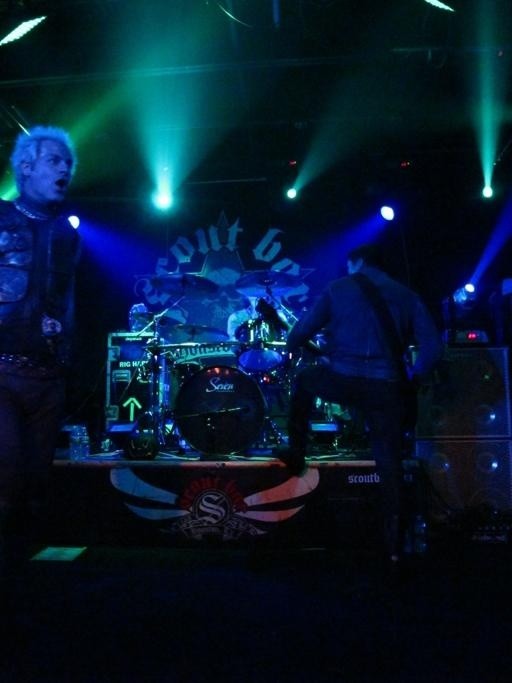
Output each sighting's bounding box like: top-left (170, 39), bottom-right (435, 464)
top-left (69, 425), bottom-right (92, 462)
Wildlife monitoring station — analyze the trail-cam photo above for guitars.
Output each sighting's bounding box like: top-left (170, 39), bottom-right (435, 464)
top-left (256, 298), bottom-right (419, 432)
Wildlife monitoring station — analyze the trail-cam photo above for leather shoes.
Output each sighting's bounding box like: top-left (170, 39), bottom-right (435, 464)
top-left (272, 446), bottom-right (306, 477)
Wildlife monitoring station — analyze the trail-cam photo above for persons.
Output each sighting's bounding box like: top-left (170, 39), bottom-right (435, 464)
top-left (272, 243), bottom-right (444, 559)
top-left (0, 125), bottom-right (93, 500)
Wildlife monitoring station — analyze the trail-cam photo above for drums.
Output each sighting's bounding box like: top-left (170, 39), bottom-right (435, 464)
top-left (168, 341), bottom-right (207, 379)
top-left (174, 365), bottom-right (268, 457)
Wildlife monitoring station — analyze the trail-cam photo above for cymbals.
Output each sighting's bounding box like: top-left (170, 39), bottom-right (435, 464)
top-left (234, 271), bottom-right (308, 296)
top-left (237, 319), bottom-right (281, 372)
top-left (150, 273), bottom-right (220, 300)
top-left (161, 324), bottom-right (229, 344)
